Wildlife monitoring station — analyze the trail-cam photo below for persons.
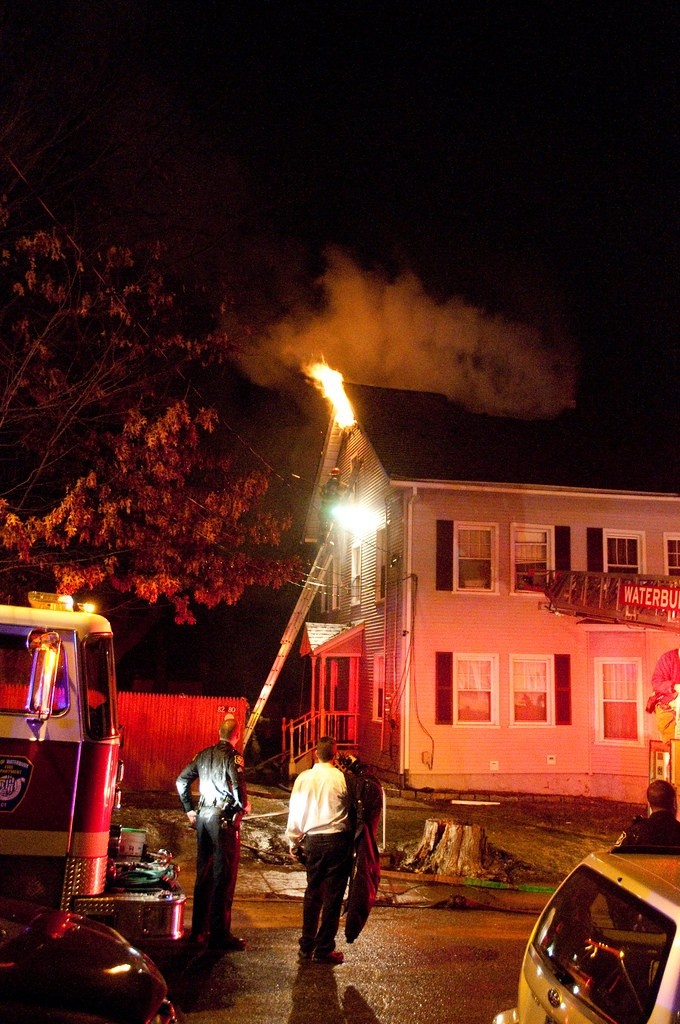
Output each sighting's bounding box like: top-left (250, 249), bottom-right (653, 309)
top-left (175, 718), bottom-right (251, 949)
top-left (652, 648), bottom-right (680, 744)
top-left (611, 780), bottom-right (680, 856)
top-left (285, 737), bottom-right (382, 961)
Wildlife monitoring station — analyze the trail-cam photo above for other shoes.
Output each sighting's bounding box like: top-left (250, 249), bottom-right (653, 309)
top-left (299, 947), bottom-right (314, 958)
top-left (313, 950), bottom-right (343, 962)
top-left (208, 931), bottom-right (245, 948)
top-left (190, 928), bottom-right (211, 944)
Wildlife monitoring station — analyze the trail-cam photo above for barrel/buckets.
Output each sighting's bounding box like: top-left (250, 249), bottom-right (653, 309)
top-left (120, 828), bottom-right (146, 862)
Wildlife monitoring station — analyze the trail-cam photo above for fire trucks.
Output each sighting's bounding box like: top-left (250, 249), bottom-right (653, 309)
top-left (648, 683), bottom-right (680, 821)
top-left (0, 591), bottom-right (187, 944)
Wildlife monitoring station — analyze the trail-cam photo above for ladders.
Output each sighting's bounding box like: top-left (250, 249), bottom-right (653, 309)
top-left (241, 455), bottom-right (365, 753)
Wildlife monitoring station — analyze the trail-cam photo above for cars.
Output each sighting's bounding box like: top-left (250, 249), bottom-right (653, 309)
top-left (490, 849), bottom-right (679, 1023)
top-left (0, 894), bottom-right (179, 1024)
